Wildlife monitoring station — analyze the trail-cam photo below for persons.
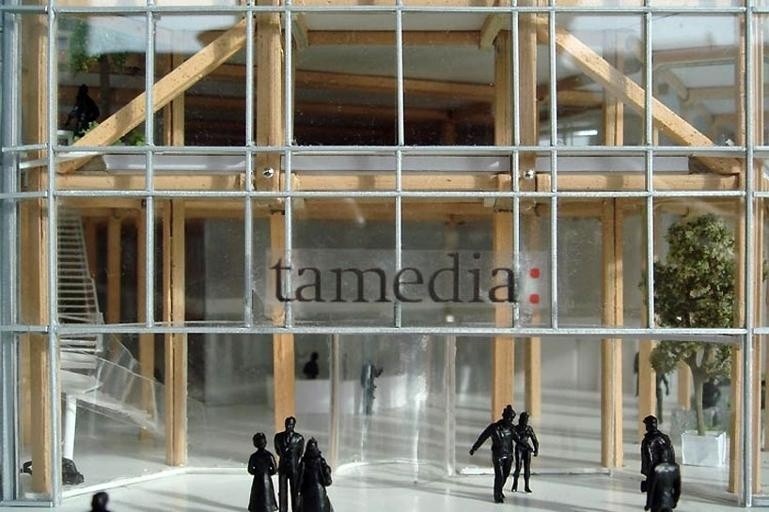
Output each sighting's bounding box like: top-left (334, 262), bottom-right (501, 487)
top-left (359, 355), bottom-right (384, 414)
top-left (248, 431), bottom-right (280, 511)
top-left (641, 415), bottom-right (676, 494)
top-left (761, 379), bottom-right (766, 452)
top-left (293, 436), bottom-right (334, 511)
top-left (702, 376), bottom-right (721, 426)
top-left (273, 415), bottom-right (306, 511)
top-left (62, 83), bottom-right (101, 141)
top-left (469, 404), bottom-right (533, 503)
top-left (512, 410), bottom-right (540, 493)
top-left (88, 491), bottom-right (113, 512)
top-left (301, 349), bottom-right (321, 380)
top-left (644, 445), bottom-right (682, 511)
top-left (632, 349), bottom-right (639, 399)
top-left (655, 368), bottom-right (670, 424)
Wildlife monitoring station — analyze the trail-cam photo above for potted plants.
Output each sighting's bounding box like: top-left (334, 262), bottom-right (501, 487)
top-left (636, 210), bottom-right (732, 470)
